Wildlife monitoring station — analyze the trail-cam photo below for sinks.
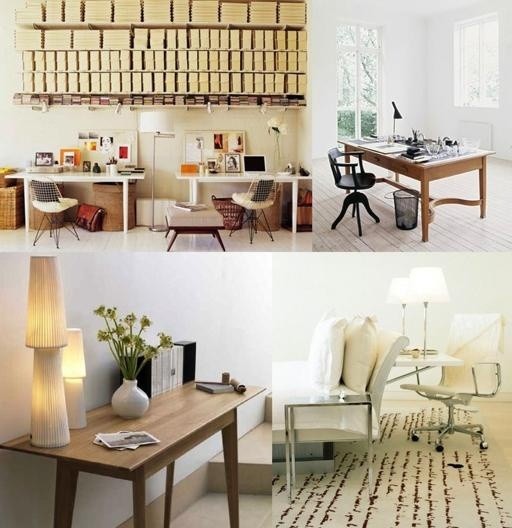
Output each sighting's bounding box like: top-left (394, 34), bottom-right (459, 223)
top-left (275, 171), bottom-right (312, 182)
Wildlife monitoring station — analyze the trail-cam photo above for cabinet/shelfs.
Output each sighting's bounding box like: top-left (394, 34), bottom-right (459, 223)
top-left (14, 20), bottom-right (307, 106)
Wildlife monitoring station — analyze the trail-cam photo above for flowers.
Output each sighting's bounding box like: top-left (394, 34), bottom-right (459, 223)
top-left (267, 118), bottom-right (287, 135)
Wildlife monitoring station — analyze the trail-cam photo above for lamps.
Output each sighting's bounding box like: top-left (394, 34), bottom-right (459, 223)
top-left (139, 112), bottom-right (174, 232)
top-left (25, 255), bottom-right (70, 448)
top-left (391, 101), bottom-right (403, 134)
top-left (408, 266), bottom-right (450, 358)
top-left (386, 277), bottom-right (416, 350)
top-left (60, 328), bottom-right (88, 429)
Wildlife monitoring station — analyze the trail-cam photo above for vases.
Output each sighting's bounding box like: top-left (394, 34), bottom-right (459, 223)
top-left (112, 378), bottom-right (149, 418)
top-left (273, 136), bottom-right (281, 173)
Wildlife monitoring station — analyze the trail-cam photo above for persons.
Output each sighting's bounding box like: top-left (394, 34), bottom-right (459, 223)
top-left (228, 157), bottom-right (236, 170)
top-left (100, 137), bottom-right (113, 153)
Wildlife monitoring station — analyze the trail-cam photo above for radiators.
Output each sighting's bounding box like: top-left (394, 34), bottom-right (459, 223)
top-left (457, 120), bottom-right (493, 151)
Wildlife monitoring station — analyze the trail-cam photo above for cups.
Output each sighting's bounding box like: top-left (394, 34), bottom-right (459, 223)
top-left (199, 162), bottom-right (209, 177)
top-left (425, 144), bottom-right (440, 159)
top-left (106, 162), bottom-right (117, 174)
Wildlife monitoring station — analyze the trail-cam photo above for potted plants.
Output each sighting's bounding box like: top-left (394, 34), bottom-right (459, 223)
top-left (94, 304), bottom-right (173, 381)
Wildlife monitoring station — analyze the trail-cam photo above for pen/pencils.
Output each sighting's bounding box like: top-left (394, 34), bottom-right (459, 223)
top-left (109, 156), bottom-right (117, 163)
top-left (412, 129), bottom-right (417, 141)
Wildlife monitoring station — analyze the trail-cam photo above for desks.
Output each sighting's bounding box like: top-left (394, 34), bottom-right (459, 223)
top-left (176, 172), bottom-right (312, 233)
top-left (165, 202), bottom-right (226, 252)
top-left (337, 134), bottom-right (496, 242)
top-left (0, 379), bottom-right (267, 528)
top-left (385, 346), bottom-right (464, 385)
top-left (5, 169), bottom-right (145, 233)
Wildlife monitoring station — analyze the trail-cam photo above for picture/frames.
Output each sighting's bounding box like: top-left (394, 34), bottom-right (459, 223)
top-left (225, 154), bottom-right (242, 173)
top-left (183, 130), bottom-right (246, 172)
top-left (116, 144), bottom-right (130, 163)
top-left (35, 151), bottom-right (53, 166)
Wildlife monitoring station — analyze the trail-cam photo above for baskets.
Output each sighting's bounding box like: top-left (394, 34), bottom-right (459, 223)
top-left (212, 194), bottom-right (243, 230)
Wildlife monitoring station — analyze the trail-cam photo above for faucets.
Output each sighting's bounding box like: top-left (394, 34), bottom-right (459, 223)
top-left (285, 164), bottom-right (291, 169)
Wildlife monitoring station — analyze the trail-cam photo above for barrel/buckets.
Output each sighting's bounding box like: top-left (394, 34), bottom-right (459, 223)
top-left (249, 183), bottom-right (283, 231)
top-left (92, 182), bottom-right (139, 232)
top-left (29, 182), bottom-right (64, 230)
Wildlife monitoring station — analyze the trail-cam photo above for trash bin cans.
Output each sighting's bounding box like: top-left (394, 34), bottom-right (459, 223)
top-left (394, 189), bottom-right (419, 230)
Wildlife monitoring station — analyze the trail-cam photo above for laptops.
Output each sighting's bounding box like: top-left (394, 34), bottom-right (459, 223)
top-left (243, 155), bottom-right (266, 176)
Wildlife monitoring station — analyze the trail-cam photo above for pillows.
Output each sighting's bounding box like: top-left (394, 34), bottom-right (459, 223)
top-left (306, 312), bottom-right (380, 393)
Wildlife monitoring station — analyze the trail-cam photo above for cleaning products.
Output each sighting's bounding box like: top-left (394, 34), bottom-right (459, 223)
top-left (93, 162), bottom-right (100, 173)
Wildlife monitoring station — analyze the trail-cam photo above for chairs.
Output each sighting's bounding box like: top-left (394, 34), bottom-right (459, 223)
top-left (327, 147), bottom-right (380, 236)
top-left (26, 176), bottom-right (80, 248)
top-left (229, 174), bottom-right (276, 244)
top-left (400, 313), bottom-right (504, 452)
top-left (272, 308), bottom-right (410, 500)
